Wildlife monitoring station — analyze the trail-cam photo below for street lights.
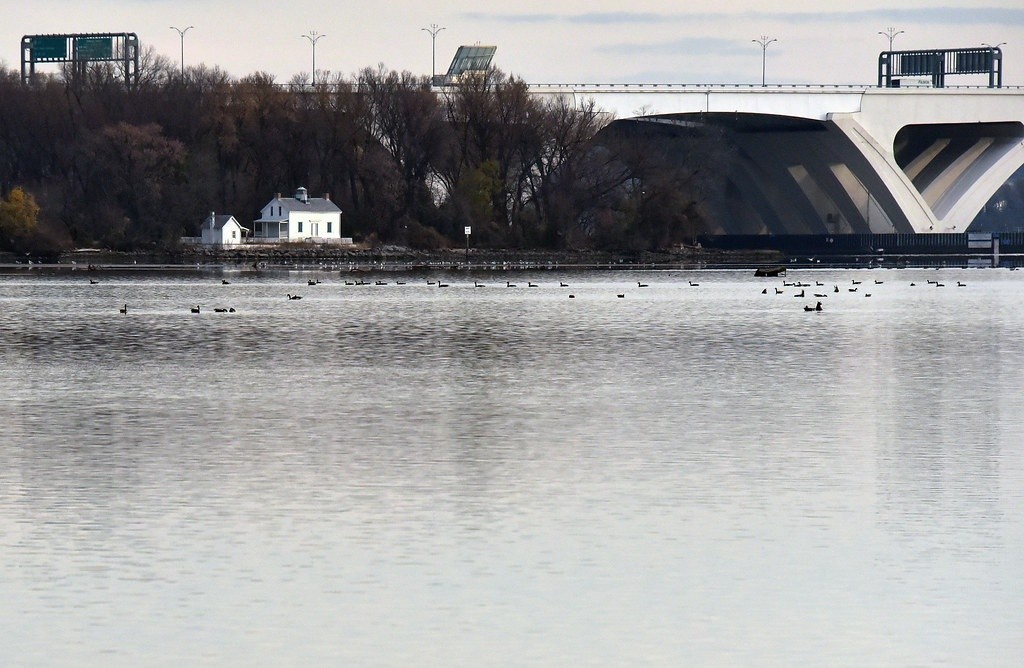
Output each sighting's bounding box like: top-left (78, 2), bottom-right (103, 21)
top-left (169, 26), bottom-right (194, 86)
top-left (421, 23), bottom-right (446, 77)
top-left (301, 30), bottom-right (326, 85)
top-left (752, 35), bottom-right (778, 87)
top-left (877, 27), bottom-right (904, 51)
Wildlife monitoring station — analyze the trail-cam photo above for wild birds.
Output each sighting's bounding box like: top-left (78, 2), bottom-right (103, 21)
top-left (689, 280), bottom-right (699, 286)
top-left (560, 282), bottom-right (568, 287)
top-left (617, 294), bottom-right (625, 298)
top-left (120, 279), bottom-right (538, 313)
top-left (16, 252), bottom-right (202, 271)
top-left (90, 279), bottom-right (99, 284)
top-left (762, 280), bottom-right (966, 312)
top-left (637, 282), bottom-right (648, 287)
